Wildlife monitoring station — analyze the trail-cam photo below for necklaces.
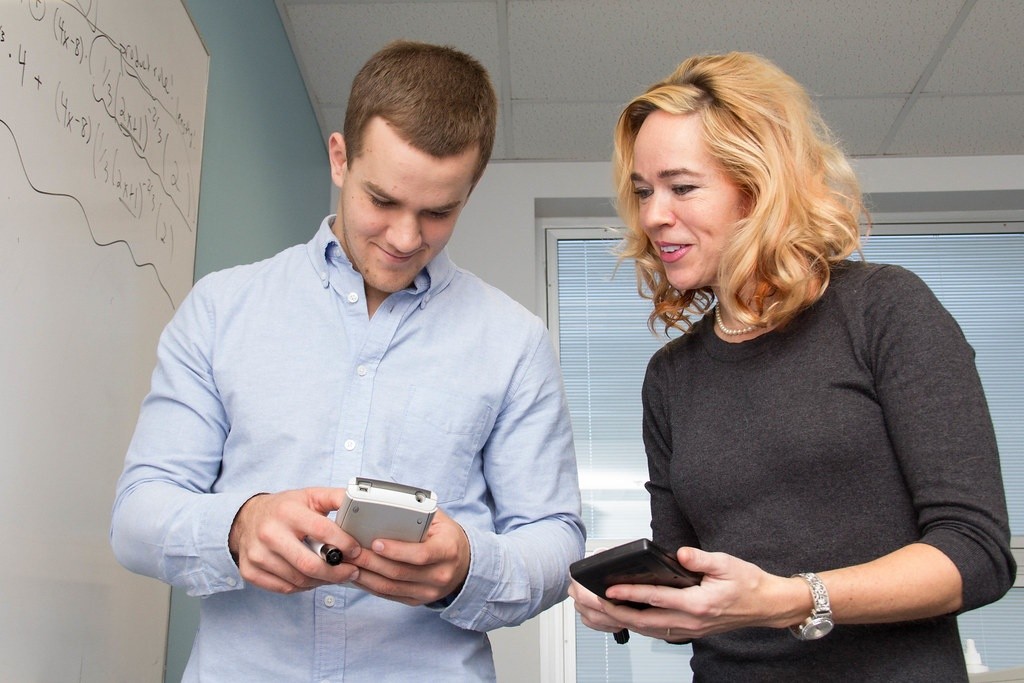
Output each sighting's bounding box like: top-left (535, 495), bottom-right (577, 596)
top-left (715, 294), bottom-right (791, 338)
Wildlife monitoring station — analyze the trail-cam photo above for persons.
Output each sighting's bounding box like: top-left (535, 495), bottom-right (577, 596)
top-left (569, 50), bottom-right (1018, 683)
top-left (109, 41), bottom-right (588, 683)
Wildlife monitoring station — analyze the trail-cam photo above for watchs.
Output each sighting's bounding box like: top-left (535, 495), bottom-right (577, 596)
top-left (786, 571), bottom-right (835, 643)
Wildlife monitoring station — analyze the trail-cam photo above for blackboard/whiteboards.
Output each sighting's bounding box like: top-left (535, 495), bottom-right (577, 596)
top-left (2, 1), bottom-right (213, 683)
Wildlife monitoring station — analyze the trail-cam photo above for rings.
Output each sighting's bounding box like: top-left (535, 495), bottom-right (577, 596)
top-left (664, 628), bottom-right (673, 640)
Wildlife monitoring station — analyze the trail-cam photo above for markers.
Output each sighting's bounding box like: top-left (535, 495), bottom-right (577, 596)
top-left (306, 539), bottom-right (343, 566)
top-left (613, 628), bottom-right (630, 645)
